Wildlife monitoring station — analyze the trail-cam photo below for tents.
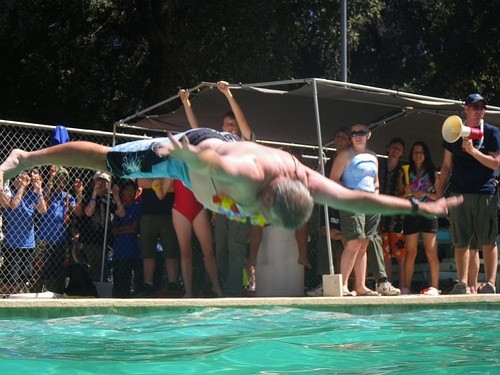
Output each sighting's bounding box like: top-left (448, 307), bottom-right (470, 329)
top-left (101, 79), bottom-right (500, 275)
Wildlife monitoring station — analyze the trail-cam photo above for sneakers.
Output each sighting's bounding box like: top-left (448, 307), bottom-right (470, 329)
top-left (422, 286), bottom-right (438, 295)
top-left (306, 283), bottom-right (324, 297)
top-left (378, 280), bottom-right (401, 296)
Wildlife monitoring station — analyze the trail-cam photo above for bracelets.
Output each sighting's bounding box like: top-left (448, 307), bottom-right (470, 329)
top-left (37, 194), bottom-right (43, 198)
top-left (408, 196), bottom-right (418, 215)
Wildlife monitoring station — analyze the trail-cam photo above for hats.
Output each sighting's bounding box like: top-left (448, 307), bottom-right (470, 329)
top-left (466, 93), bottom-right (488, 107)
top-left (57, 167), bottom-right (69, 181)
top-left (93, 171), bottom-right (109, 182)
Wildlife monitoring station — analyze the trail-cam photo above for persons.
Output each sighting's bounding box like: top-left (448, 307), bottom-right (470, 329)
top-left (437, 94), bottom-right (500, 294)
top-left (0, 128), bottom-right (463, 229)
top-left (137, 81), bottom-right (312, 297)
top-left (306, 123), bottom-right (439, 296)
top-left (0, 165), bottom-right (141, 297)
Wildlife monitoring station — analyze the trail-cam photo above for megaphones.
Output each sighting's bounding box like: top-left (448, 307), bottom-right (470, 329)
top-left (441, 115), bottom-right (483, 143)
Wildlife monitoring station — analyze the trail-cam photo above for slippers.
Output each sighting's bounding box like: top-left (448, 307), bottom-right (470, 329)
top-left (342, 290), bottom-right (353, 296)
top-left (357, 290), bottom-right (378, 296)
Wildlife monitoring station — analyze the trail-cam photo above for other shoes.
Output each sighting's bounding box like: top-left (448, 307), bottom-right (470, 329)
top-left (169, 282), bottom-right (182, 297)
top-left (134, 285), bottom-right (152, 298)
top-left (479, 282), bottom-right (497, 292)
top-left (451, 282), bottom-right (467, 294)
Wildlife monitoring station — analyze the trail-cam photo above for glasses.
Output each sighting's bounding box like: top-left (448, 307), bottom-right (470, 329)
top-left (469, 104), bottom-right (486, 111)
top-left (350, 130), bottom-right (369, 137)
